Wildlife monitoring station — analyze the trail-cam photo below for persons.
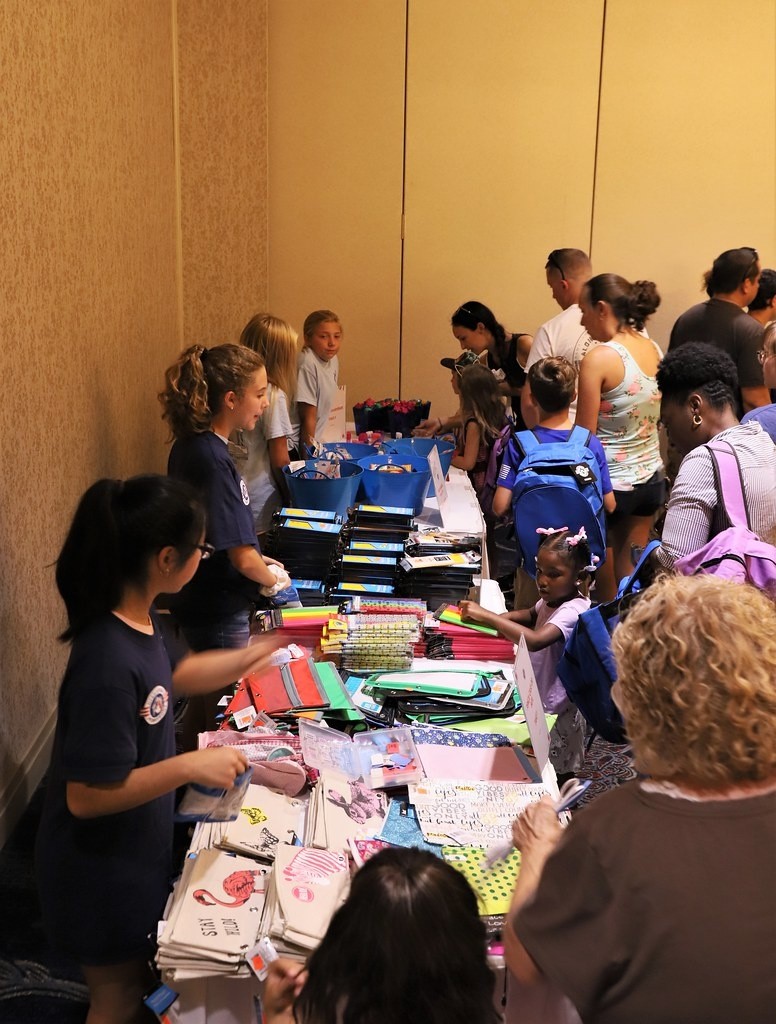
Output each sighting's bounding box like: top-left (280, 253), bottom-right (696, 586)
top-left (505, 571), bottom-right (775, 1024)
top-left (162, 341), bottom-right (285, 718)
top-left (230, 318), bottom-right (300, 547)
top-left (51, 473), bottom-right (294, 1022)
top-left (430, 243), bottom-right (776, 777)
top-left (262, 845), bottom-right (508, 1023)
top-left (295, 308), bottom-right (344, 455)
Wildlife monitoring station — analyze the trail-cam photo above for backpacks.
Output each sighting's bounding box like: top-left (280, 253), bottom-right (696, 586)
top-left (512, 423), bottom-right (606, 580)
top-left (463, 412), bottom-right (515, 540)
top-left (672, 439), bottom-right (776, 602)
top-left (556, 539), bottom-right (662, 753)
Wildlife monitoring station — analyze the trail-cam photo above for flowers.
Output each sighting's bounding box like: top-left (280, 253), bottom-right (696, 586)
top-left (355, 398), bottom-right (418, 414)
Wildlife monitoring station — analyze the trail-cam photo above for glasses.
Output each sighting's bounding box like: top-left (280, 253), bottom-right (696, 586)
top-left (740, 246), bottom-right (758, 282)
top-left (548, 249), bottom-right (565, 289)
top-left (757, 349), bottom-right (773, 364)
top-left (454, 306), bottom-right (471, 314)
top-left (157, 537), bottom-right (216, 561)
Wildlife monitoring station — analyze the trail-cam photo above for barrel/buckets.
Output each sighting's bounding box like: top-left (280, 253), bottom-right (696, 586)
top-left (357, 454), bottom-right (432, 516)
top-left (382, 433), bottom-right (456, 498)
top-left (303, 439), bottom-right (383, 464)
top-left (282, 450), bottom-right (364, 524)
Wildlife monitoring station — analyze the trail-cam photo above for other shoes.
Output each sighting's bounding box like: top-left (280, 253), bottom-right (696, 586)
top-left (497, 574), bottom-right (515, 593)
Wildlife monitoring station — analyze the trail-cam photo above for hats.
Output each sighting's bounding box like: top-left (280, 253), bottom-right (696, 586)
top-left (440, 352), bottom-right (482, 372)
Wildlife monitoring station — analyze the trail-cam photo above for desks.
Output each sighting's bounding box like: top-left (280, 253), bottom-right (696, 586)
top-left (346, 421), bottom-right (491, 580)
top-left (161, 579), bottom-right (582, 1024)
